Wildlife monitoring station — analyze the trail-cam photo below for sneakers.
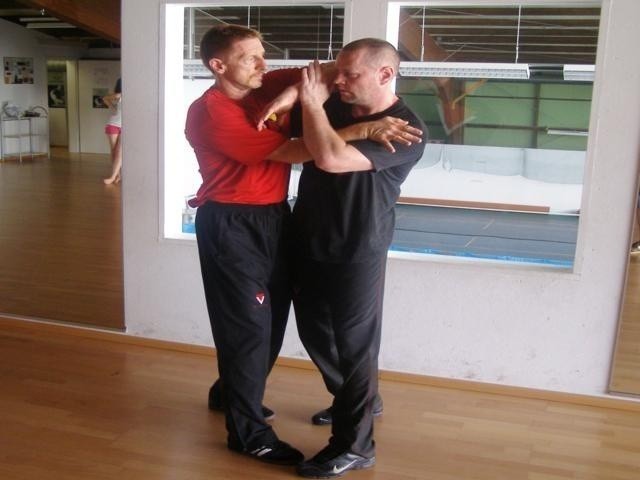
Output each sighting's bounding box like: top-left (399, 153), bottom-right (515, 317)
top-left (209, 391), bottom-right (275, 421)
top-left (312, 398), bottom-right (384, 425)
top-left (297, 444), bottom-right (376, 479)
top-left (228, 435), bottom-right (304, 465)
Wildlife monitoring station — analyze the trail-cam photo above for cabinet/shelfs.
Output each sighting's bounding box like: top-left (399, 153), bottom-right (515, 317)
top-left (0, 105), bottom-right (51, 163)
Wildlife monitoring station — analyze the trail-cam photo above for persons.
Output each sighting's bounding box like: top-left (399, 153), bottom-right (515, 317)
top-left (278, 38), bottom-right (430, 479)
top-left (102, 77), bottom-right (123, 185)
top-left (182, 24), bottom-right (424, 467)
